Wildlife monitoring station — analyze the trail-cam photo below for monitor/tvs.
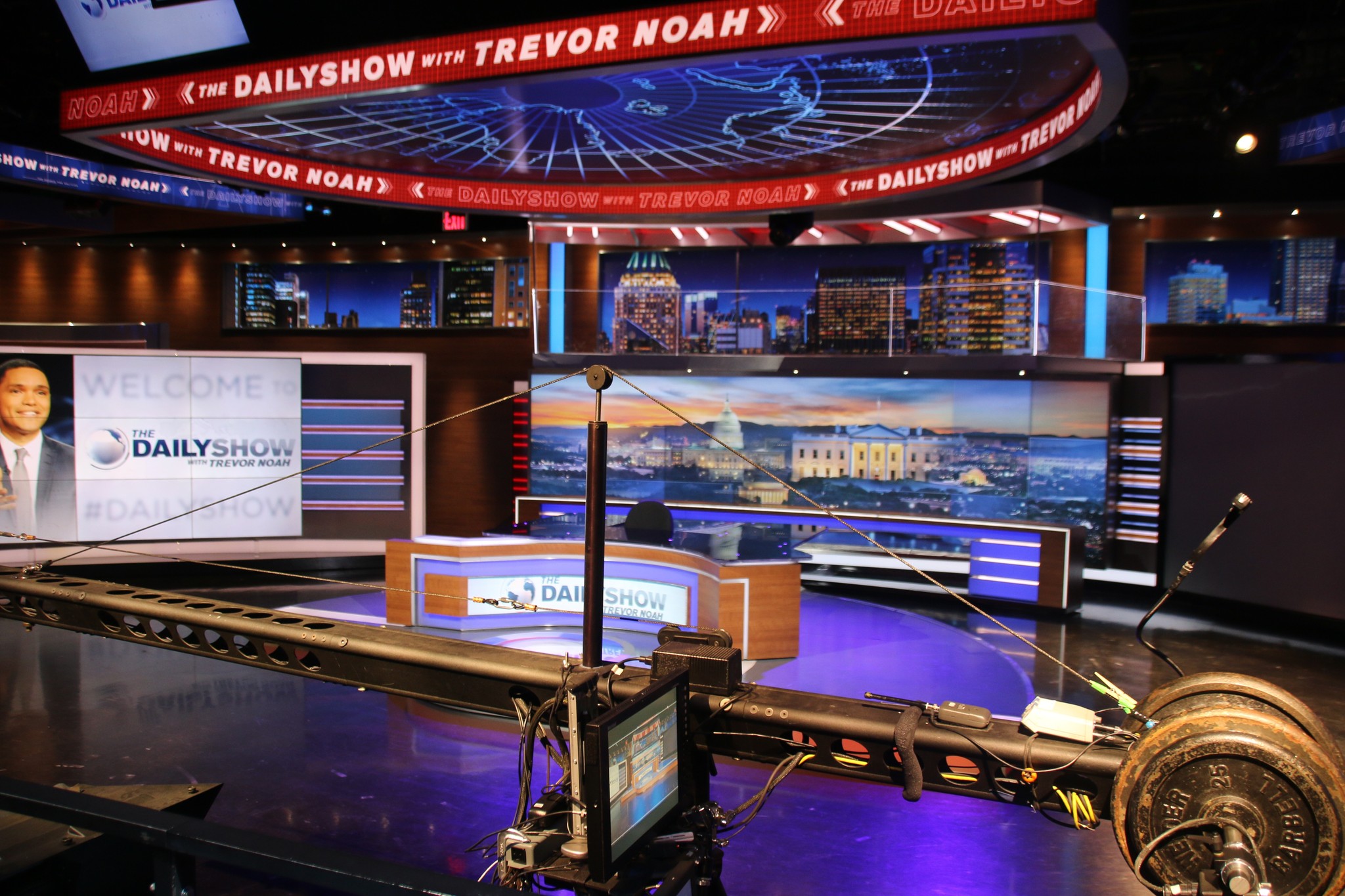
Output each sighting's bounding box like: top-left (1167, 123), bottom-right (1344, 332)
top-left (51, 0), bottom-right (258, 83)
top-left (583, 667), bottom-right (696, 883)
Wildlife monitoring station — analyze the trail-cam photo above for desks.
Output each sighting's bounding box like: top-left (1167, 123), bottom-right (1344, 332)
top-left (385, 538), bottom-right (812, 659)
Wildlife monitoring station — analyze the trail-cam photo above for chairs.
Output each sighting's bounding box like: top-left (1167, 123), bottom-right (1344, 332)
top-left (608, 500), bottom-right (674, 532)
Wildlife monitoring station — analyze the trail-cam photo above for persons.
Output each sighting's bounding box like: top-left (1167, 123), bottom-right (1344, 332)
top-left (0, 359), bottom-right (77, 543)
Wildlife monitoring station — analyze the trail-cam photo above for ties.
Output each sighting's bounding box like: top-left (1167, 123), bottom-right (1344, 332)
top-left (12, 448), bottom-right (32, 536)
